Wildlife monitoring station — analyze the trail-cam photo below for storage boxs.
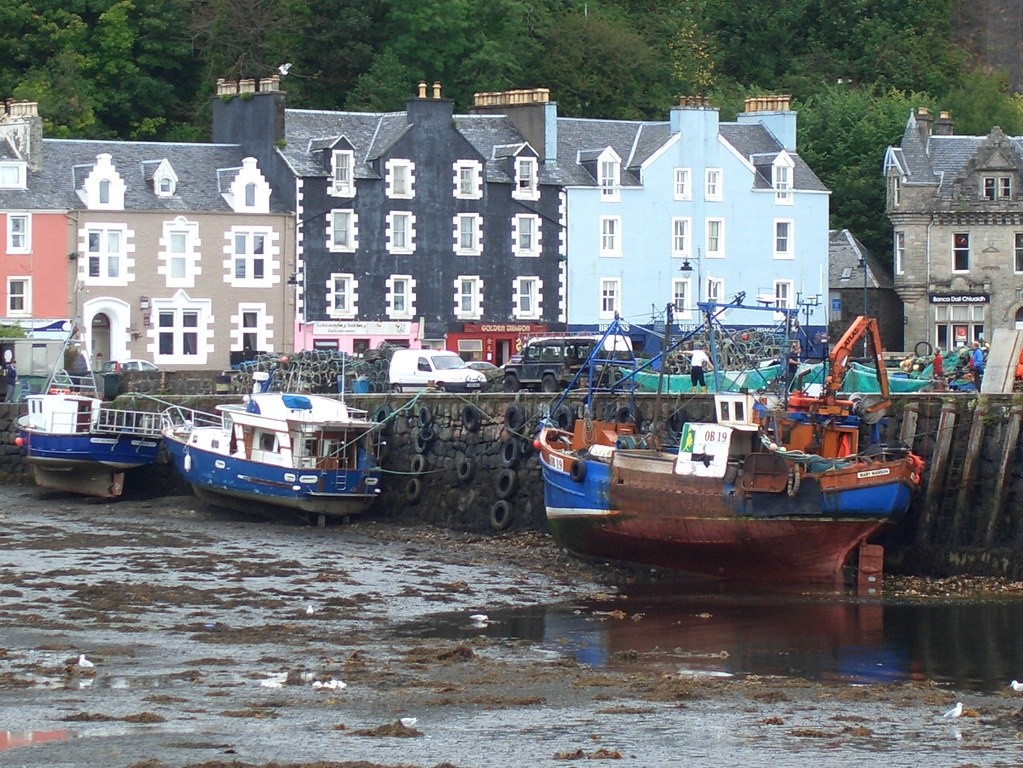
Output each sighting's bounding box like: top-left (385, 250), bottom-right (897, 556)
top-left (352, 378), bottom-right (370, 393)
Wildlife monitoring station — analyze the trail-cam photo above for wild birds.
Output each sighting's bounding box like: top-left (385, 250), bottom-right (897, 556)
top-left (1010, 680), bottom-right (1023, 691)
top-left (78, 654), bottom-right (94, 667)
top-left (943, 702), bottom-right (963, 717)
top-left (469, 614), bottom-right (488, 621)
top-left (401, 718), bottom-right (417, 726)
top-left (306, 604), bottom-right (313, 613)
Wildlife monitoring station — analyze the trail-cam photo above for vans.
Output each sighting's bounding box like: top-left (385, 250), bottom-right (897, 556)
top-left (388, 347), bottom-right (486, 393)
top-left (523, 334), bottom-right (634, 352)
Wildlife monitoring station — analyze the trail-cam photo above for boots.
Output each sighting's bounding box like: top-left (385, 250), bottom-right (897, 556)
top-left (702, 386), bottom-right (707, 394)
top-left (693, 386), bottom-right (696, 393)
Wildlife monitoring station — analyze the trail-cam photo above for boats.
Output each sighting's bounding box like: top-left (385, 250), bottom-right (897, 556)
top-left (11, 316), bottom-right (166, 500)
top-left (159, 372), bottom-right (387, 526)
top-left (535, 297), bottom-right (922, 595)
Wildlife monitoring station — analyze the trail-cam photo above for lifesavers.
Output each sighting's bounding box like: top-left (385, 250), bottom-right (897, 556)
top-left (494, 469), bottom-right (519, 499)
top-left (521, 439), bottom-right (531, 455)
top-left (457, 458), bottom-right (474, 482)
top-left (504, 405), bottom-right (526, 434)
top-left (615, 406), bottom-right (642, 430)
top-left (418, 406), bottom-right (432, 429)
top-left (490, 499), bottom-right (513, 530)
top-left (666, 408), bottom-right (688, 439)
top-left (410, 454), bottom-right (428, 478)
top-left (570, 459), bottom-right (587, 482)
top-left (374, 404), bottom-right (393, 430)
top-left (415, 432), bottom-right (429, 453)
top-left (550, 404), bottom-right (575, 432)
top-left (420, 428), bottom-right (435, 441)
top-left (406, 479), bottom-right (422, 502)
top-left (788, 464), bottom-right (801, 498)
top-left (461, 404), bottom-right (481, 432)
top-left (48, 388), bottom-right (70, 396)
top-left (500, 437), bottom-right (522, 468)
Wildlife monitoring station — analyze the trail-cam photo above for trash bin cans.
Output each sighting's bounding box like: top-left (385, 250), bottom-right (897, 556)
top-left (102, 373), bottom-right (120, 400)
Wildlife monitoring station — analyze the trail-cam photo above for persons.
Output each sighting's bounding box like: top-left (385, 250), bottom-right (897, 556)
top-left (679, 346), bottom-right (714, 394)
top-left (932, 347), bottom-right (943, 380)
top-left (787, 347), bottom-right (801, 392)
top-left (970, 341), bottom-right (984, 392)
top-left (4, 359), bottom-right (17, 403)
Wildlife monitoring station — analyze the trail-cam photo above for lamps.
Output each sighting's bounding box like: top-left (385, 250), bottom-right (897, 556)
top-left (68, 253), bottom-right (79, 261)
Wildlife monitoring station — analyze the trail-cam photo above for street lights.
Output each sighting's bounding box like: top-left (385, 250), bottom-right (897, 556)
top-left (857, 257), bottom-right (868, 360)
top-left (678, 256), bottom-right (702, 328)
top-left (288, 272), bottom-right (307, 351)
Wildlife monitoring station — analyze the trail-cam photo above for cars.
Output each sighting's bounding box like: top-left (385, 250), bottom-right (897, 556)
top-left (453, 362), bottom-right (497, 370)
top-left (101, 360), bottom-right (159, 371)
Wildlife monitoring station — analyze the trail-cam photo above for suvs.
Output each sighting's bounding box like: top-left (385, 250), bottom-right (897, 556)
top-left (503, 339), bottom-right (610, 393)
top-left (0, 338), bottom-right (88, 395)
